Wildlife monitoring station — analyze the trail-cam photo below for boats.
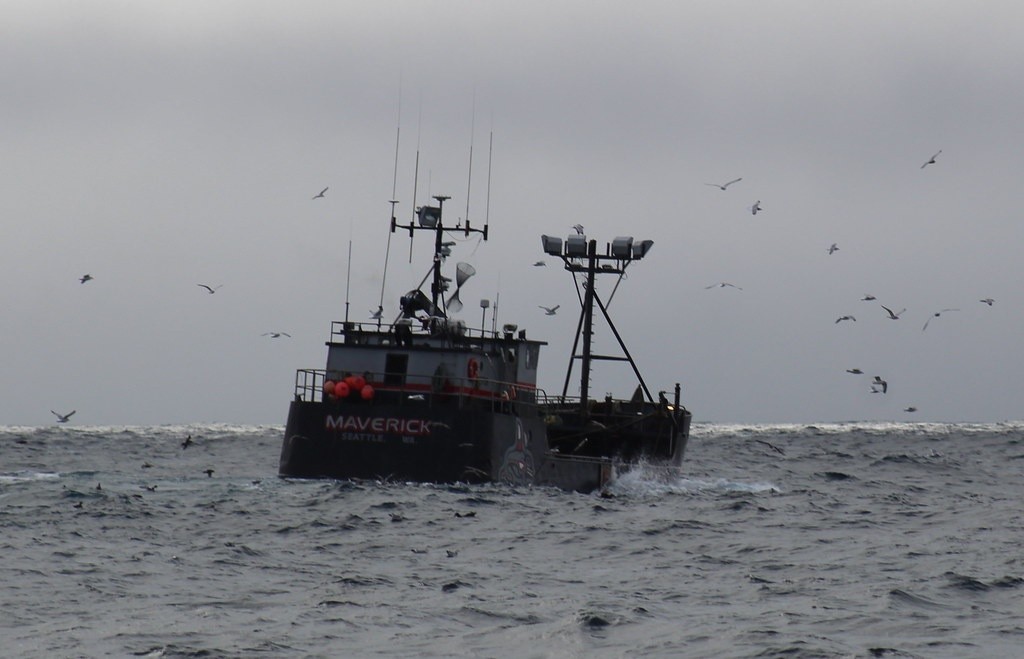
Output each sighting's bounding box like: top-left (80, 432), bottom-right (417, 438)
top-left (277, 126), bottom-right (552, 482)
top-left (533, 451), bottom-right (617, 491)
top-left (531, 232), bottom-right (693, 471)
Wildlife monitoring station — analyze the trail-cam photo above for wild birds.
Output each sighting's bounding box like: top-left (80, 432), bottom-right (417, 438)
top-left (904, 407), bottom-right (917, 413)
top-left (704, 283), bottom-right (742, 290)
top-left (980, 298), bottom-right (995, 306)
top-left (533, 260), bottom-right (545, 266)
top-left (880, 304), bottom-right (907, 320)
top-left (538, 305), bottom-right (560, 315)
top-left (76, 432), bottom-right (216, 509)
top-left (827, 244), bottom-right (840, 255)
top-left (369, 306), bottom-right (384, 319)
top-left (846, 369), bottom-right (888, 394)
top-left (920, 149), bottom-right (942, 168)
top-left (261, 331), bottom-right (291, 338)
top-left (312, 187), bottom-right (328, 199)
top-left (861, 295), bottom-right (876, 301)
top-left (836, 315), bottom-right (856, 323)
top-left (572, 224), bottom-right (584, 235)
top-left (199, 282), bottom-right (222, 295)
top-left (922, 307), bottom-right (961, 331)
top-left (52, 409), bottom-right (74, 419)
top-left (80, 274), bottom-right (93, 284)
top-left (706, 177), bottom-right (762, 215)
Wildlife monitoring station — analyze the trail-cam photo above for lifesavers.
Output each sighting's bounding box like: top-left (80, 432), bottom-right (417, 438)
top-left (467, 358), bottom-right (480, 381)
